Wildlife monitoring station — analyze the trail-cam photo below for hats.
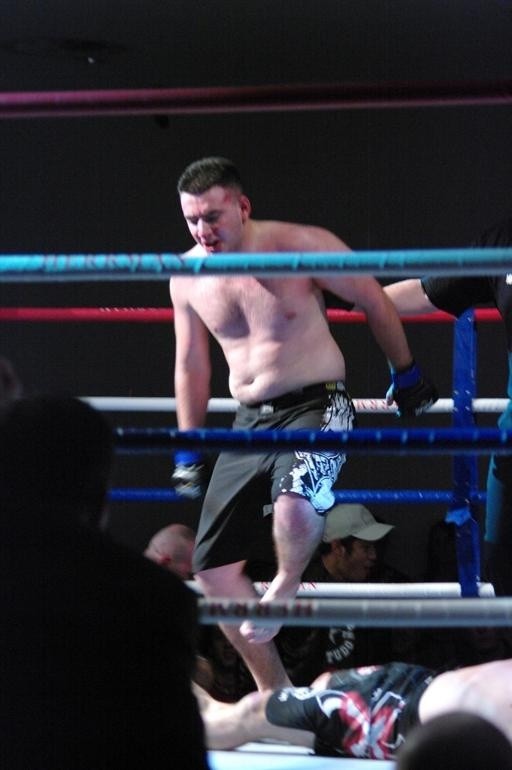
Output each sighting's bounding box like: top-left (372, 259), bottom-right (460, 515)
top-left (321, 502), bottom-right (398, 544)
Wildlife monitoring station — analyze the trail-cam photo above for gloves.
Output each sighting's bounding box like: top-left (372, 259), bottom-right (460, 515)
top-left (384, 358), bottom-right (440, 423)
top-left (168, 442), bottom-right (212, 501)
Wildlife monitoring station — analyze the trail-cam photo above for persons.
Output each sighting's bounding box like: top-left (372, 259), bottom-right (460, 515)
top-left (166, 158), bottom-right (435, 693)
top-left (367, 270), bottom-right (511, 603)
